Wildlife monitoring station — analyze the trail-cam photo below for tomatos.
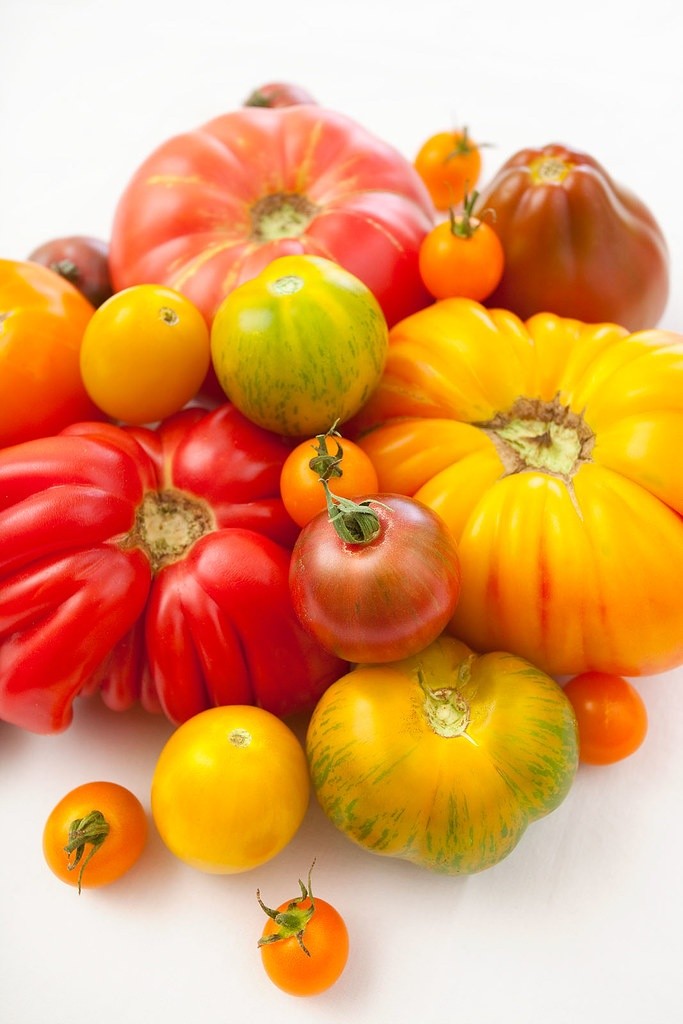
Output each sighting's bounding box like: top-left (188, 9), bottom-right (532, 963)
top-left (0, 84), bottom-right (683, 998)
